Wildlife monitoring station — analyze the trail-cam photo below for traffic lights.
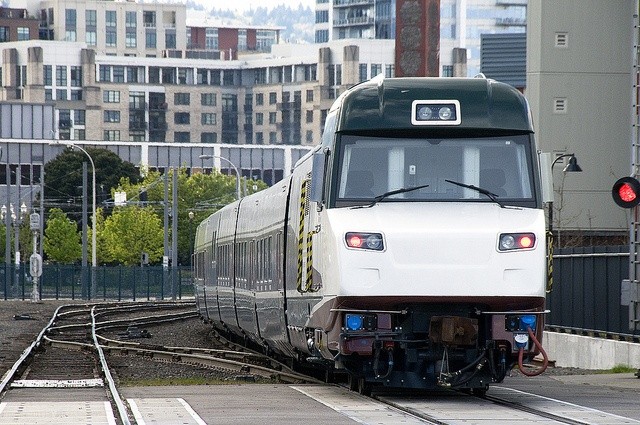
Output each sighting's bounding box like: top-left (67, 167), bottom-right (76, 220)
top-left (612, 176), bottom-right (640, 208)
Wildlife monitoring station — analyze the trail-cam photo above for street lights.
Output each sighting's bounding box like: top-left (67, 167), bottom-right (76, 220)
top-left (50, 141), bottom-right (101, 298)
top-left (199, 154), bottom-right (241, 200)
top-left (545, 153), bottom-right (583, 325)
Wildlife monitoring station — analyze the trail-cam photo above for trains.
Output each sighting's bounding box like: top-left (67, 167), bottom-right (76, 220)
top-left (192, 73), bottom-right (552, 397)
